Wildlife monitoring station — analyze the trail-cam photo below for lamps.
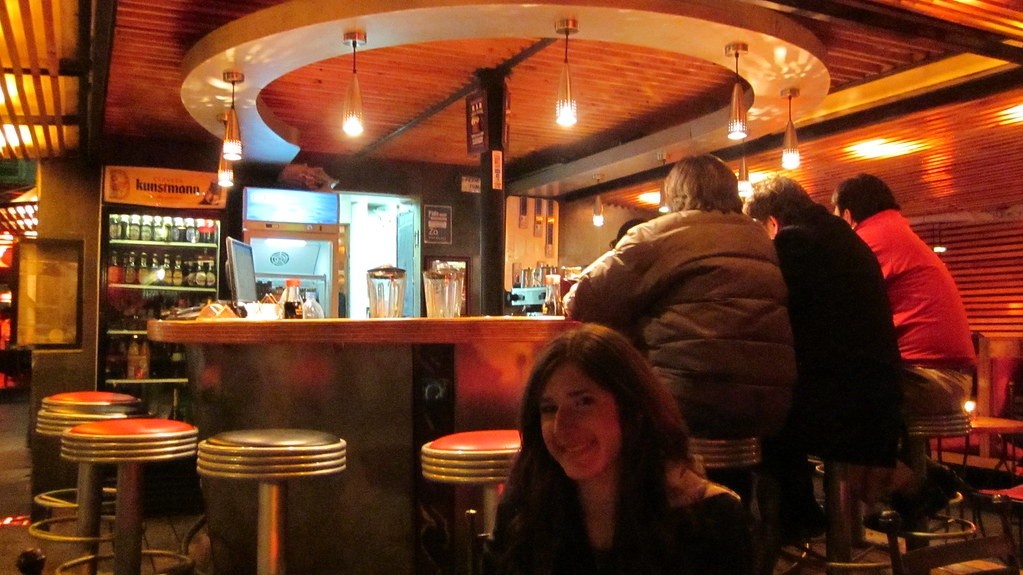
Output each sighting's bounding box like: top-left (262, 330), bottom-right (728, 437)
top-left (343, 31), bottom-right (363, 137)
top-left (737, 154), bottom-right (752, 196)
top-left (780, 86), bottom-right (801, 170)
top-left (724, 42), bottom-right (749, 141)
top-left (223, 71), bottom-right (243, 161)
top-left (658, 151), bottom-right (672, 213)
top-left (554, 18), bottom-right (580, 127)
top-left (592, 174), bottom-right (603, 226)
top-left (216, 113), bottom-right (234, 187)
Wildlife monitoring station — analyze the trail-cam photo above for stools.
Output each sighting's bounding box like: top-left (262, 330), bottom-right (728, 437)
top-left (686, 437), bottom-right (760, 490)
top-left (28, 389), bottom-right (345, 575)
top-left (904, 416), bottom-right (978, 545)
top-left (420, 429), bottom-right (524, 540)
top-left (779, 452), bottom-right (903, 574)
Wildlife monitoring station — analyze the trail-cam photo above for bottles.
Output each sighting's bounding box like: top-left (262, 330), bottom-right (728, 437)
top-left (105, 214), bottom-right (218, 379)
top-left (302, 288), bottom-right (324, 319)
top-left (283, 279), bottom-right (303, 319)
top-left (543, 274), bottom-right (565, 316)
top-left (167, 388), bottom-right (184, 423)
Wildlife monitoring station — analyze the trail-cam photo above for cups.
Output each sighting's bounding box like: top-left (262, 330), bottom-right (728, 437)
top-left (366, 264), bottom-right (407, 319)
top-left (422, 263), bottom-right (457, 318)
top-left (520, 267), bottom-right (582, 288)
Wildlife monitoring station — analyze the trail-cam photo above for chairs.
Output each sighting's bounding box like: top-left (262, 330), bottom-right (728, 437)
top-left (878, 494), bottom-right (1023, 575)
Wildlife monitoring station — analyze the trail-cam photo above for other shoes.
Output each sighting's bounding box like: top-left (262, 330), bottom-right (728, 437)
top-left (882, 463), bottom-right (964, 531)
top-left (780, 501), bottom-right (830, 548)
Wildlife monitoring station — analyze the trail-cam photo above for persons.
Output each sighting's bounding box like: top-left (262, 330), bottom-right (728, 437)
top-left (560, 154), bottom-right (798, 446)
top-left (485, 322), bottom-right (755, 575)
top-left (741, 171), bottom-right (905, 542)
top-left (829, 173), bottom-right (977, 532)
